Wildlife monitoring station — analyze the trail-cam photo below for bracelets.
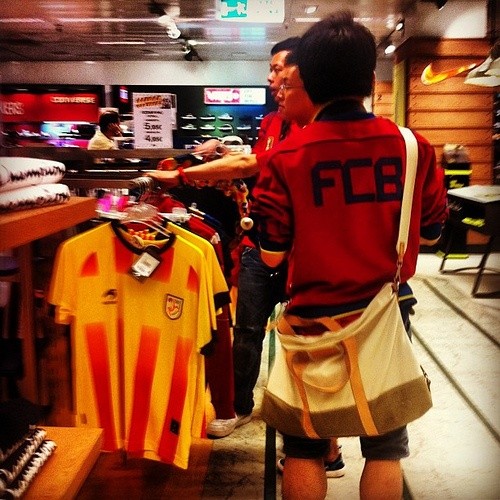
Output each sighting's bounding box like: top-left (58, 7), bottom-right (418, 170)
top-left (215, 144), bottom-right (226, 156)
top-left (178, 166), bottom-right (188, 184)
top-left (116, 132), bottom-right (120, 135)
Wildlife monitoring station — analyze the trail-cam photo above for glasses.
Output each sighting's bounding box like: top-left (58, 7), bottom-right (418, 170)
top-left (279, 84), bottom-right (304, 91)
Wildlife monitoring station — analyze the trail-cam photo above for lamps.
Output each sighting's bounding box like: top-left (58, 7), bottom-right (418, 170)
top-left (435, 0), bottom-right (447, 10)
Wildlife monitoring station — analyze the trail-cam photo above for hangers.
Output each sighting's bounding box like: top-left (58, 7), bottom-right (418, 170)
top-left (118, 176), bottom-right (206, 239)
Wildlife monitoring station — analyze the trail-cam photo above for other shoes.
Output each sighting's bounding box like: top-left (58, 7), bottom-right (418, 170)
top-left (208, 411), bottom-right (251, 436)
top-left (278, 453), bottom-right (347, 479)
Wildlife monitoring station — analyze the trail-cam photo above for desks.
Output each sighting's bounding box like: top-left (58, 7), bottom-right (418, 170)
top-left (440, 184), bottom-right (500, 296)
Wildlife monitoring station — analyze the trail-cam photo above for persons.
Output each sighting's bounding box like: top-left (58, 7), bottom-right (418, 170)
top-left (250, 10), bottom-right (449, 500)
top-left (87, 110), bottom-right (124, 164)
top-left (193, 35), bottom-right (306, 438)
top-left (141, 45), bottom-right (347, 478)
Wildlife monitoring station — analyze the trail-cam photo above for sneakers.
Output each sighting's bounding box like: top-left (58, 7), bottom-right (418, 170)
top-left (179, 113), bottom-right (267, 142)
top-left (0, 127), bottom-right (83, 148)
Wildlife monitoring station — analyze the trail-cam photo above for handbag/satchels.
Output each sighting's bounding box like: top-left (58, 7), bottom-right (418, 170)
top-left (252, 278), bottom-right (431, 439)
